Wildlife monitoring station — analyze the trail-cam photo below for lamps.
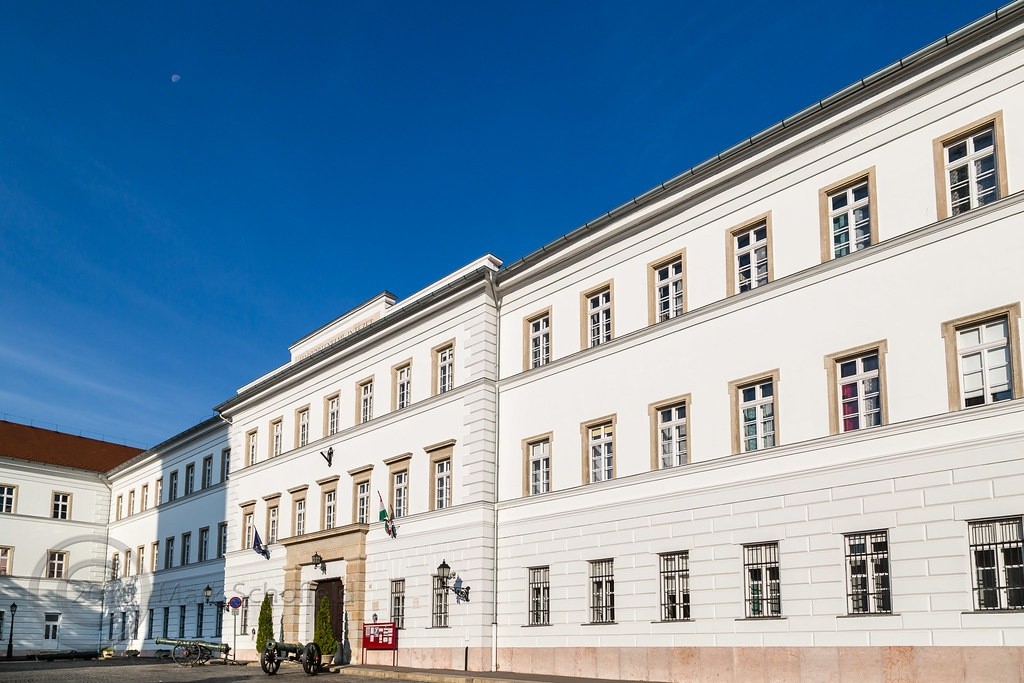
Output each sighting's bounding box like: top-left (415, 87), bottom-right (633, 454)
top-left (437, 558), bottom-right (470, 602)
top-left (204, 584), bottom-right (225, 611)
top-left (312, 551), bottom-right (326, 574)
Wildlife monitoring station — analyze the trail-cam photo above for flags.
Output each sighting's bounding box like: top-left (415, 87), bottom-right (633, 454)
top-left (253, 531), bottom-right (263, 554)
top-left (379, 497), bottom-right (391, 536)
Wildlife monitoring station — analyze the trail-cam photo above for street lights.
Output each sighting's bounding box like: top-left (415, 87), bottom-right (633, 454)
top-left (6, 601), bottom-right (18, 660)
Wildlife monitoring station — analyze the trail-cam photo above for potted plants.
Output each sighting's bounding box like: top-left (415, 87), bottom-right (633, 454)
top-left (103, 647), bottom-right (113, 657)
top-left (256, 592), bottom-right (275, 662)
top-left (155, 651), bottom-right (171, 659)
top-left (313, 596), bottom-right (338, 667)
top-left (126, 650), bottom-right (141, 660)
